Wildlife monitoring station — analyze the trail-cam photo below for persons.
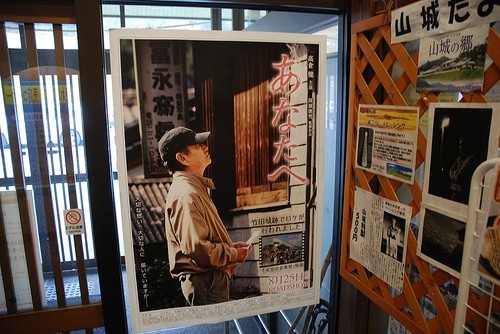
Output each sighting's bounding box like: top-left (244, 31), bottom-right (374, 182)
top-left (386, 218), bottom-right (400, 259)
top-left (451, 135), bottom-right (474, 203)
top-left (158, 126), bottom-right (252, 306)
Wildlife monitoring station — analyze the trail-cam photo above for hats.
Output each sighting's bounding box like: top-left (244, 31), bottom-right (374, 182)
top-left (158, 126), bottom-right (211, 162)
